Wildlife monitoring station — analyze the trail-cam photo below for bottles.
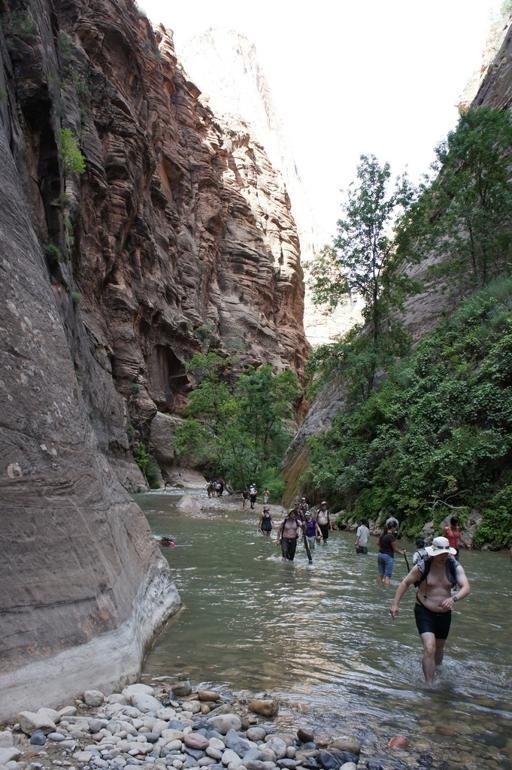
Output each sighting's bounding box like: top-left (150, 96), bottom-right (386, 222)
top-left (305, 511), bottom-right (311, 516)
top-left (425, 537), bottom-right (457, 556)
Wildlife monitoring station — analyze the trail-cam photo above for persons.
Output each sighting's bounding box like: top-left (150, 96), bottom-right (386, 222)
top-left (412, 538), bottom-right (428, 565)
top-left (276, 496), bottom-right (332, 565)
top-left (257, 505), bottom-right (275, 538)
top-left (354, 519), bottom-right (371, 554)
top-left (389, 536), bottom-right (471, 687)
top-left (242, 488), bottom-right (250, 509)
top-left (262, 487), bottom-right (271, 504)
top-left (376, 520), bottom-right (407, 585)
top-left (248, 482), bottom-right (258, 509)
top-left (384, 508), bottom-right (400, 541)
top-left (207, 479), bottom-right (225, 499)
top-left (441, 515), bottom-right (470, 591)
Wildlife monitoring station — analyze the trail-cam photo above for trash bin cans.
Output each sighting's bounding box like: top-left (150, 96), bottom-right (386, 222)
top-left (452, 595), bottom-right (458, 602)
